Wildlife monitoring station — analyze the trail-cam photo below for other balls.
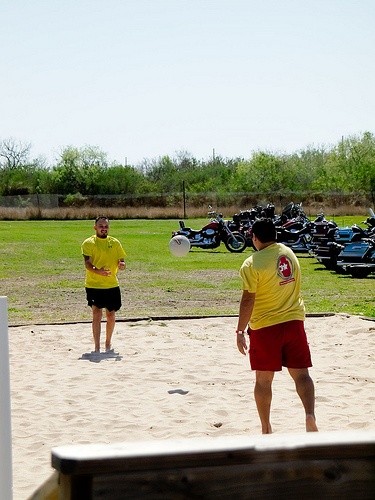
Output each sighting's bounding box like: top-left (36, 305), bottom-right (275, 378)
top-left (167, 232), bottom-right (192, 260)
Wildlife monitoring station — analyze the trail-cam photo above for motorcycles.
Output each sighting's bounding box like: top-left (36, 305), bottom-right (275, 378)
top-left (224, 202), bottom-right (375, 279)
top-left (171, 205), bottom-right (247, 253)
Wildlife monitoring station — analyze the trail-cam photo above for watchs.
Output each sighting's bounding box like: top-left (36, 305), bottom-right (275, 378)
top-left (236, 329), bottom-right (244, 336)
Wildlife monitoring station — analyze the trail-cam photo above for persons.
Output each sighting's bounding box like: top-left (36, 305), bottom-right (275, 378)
top-left (236, 220), bottom-right (318, 434)
top-left (81, 216), bottom-right (127, 358)
